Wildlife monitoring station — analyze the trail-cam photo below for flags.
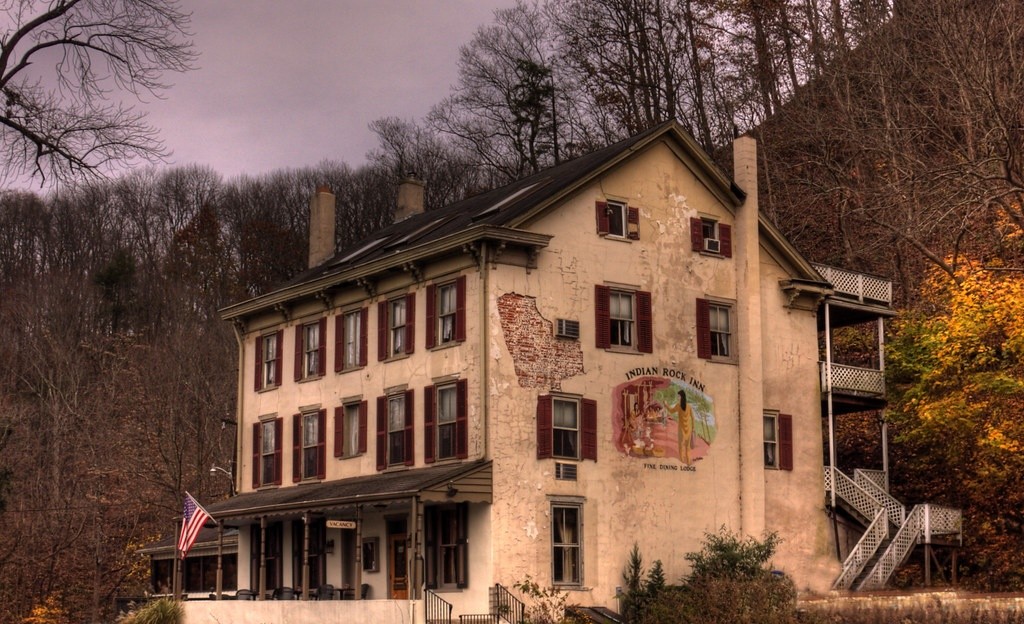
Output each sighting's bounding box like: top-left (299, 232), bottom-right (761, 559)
top-left (177, 489), bottom-right (210, 559)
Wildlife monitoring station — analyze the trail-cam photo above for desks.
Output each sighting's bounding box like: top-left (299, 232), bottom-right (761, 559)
top-left (251, 588), bottom-right (355, 600)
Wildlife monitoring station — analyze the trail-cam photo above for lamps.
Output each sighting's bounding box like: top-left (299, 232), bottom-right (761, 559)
top-left (209, 466), bottom-right (231, 481)
top-left (599, 181), bottom-right (614, 218)
top-left (446, 480), bottom-right (458, 497)
top-left (326, 539), bottom-right (334, 554)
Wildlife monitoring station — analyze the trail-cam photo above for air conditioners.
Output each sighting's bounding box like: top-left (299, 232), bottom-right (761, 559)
top-left (704, 238), bottom-right (720, 254)
top-left (554, 319), bottom-right (581, 339)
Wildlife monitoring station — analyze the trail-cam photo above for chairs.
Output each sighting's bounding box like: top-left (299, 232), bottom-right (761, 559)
top-left (312, 585), bottom-right (335, 600)
top-left (345, 583), bottom-right (369, 600)
top-left (209, 593), bottom-right (230, 600)
top-left (279, 587), bottom-right (295, 600)
top-left (236, 589), bottom-right (251, 600)
